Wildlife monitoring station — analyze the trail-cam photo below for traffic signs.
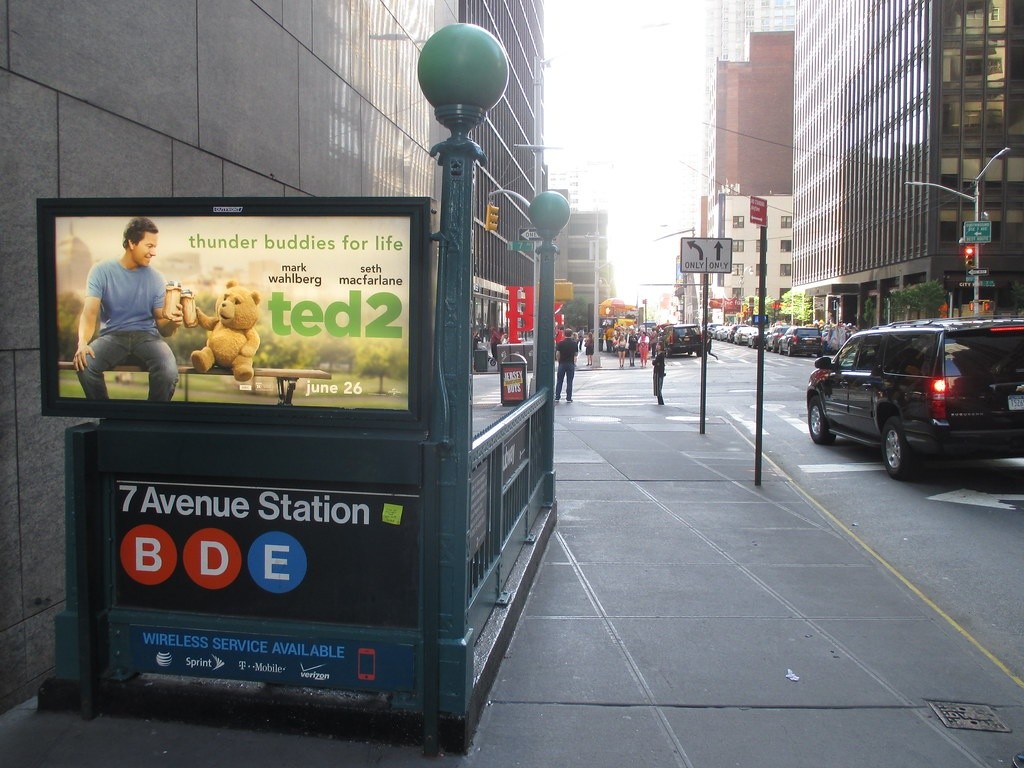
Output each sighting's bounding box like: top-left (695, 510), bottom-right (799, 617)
top-left (967, 268), bottom-right (989, 276)
top-left (518, 228), bottom-right (543, 241)
top-left (681, 236), bottom-right (733, 276)
top-left (964, 221), bottom-right (992, 243)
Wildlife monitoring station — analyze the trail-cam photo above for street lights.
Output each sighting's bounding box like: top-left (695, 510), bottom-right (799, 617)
top-left (904, 146), bottom-right (1011, 316)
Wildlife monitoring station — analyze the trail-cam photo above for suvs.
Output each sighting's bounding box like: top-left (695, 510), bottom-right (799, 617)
top-left (777, 325), bottom-right (826, 358)
top-left (806, 316), bottom-right (1024, 482)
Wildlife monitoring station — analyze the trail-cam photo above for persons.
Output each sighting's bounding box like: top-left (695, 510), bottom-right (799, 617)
top-left (652, 342), bottom-right (666, 406)
top-left (490, 327), bottom-right (500, 359)
top-left (501, 333), bottom-right (510, 344)
top-left (473, 332), bottom-right (481, 349)
top-left (573, 330), bottom-right (594, 365)
top-left (604, 326), bottom-right (657, 367)
top-left (707, 331), bottom-right (718, 360)
top-left (73, 217), bottom-right (184, 401)
top-left (555, 328), bottom-right (578, 403)
top-left (811, 320), bottom-right (860, 355)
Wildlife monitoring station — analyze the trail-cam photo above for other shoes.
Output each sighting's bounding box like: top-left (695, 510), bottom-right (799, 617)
top-left (555, 396), bottom-right (560, 401)
top-left (566, 397), bottom-right (572, 403)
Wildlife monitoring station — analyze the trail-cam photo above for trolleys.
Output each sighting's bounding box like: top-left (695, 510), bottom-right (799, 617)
top-left (476, 341), bottom-right (498, 366)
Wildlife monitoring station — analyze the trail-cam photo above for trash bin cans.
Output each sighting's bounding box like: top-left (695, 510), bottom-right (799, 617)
top-left (499, 352), bottom-right (528, 407)
top-left (474, 350), bottom-right (488, 373)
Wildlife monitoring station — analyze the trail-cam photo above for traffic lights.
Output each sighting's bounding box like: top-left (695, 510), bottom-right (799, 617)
top-left (969, 301), bottom-right (974, 312)
top-left (485, 203), bottom-right (500, 231)
top-left (982, 301), bottom-right (992, 313)
top-left (964, 247), bottom-right (974, 267)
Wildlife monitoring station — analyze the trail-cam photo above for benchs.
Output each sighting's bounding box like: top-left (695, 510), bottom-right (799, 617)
top-left (57, 361), bottom-right (332, 406)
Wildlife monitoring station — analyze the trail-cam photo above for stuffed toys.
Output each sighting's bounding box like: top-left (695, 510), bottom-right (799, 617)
top-left (191, 280), bottom-right (261, 382)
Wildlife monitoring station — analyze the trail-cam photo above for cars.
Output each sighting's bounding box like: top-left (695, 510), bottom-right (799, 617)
top-left (660, 324), bottom-right (713, 359)
top-left (643, 321), bottom-right (791, 353)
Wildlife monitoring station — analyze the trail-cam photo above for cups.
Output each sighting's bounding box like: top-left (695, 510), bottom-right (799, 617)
top-left (181, 295), bottom-right (198, 328)
top-left (162, 286), bottom-right (182, 321)
